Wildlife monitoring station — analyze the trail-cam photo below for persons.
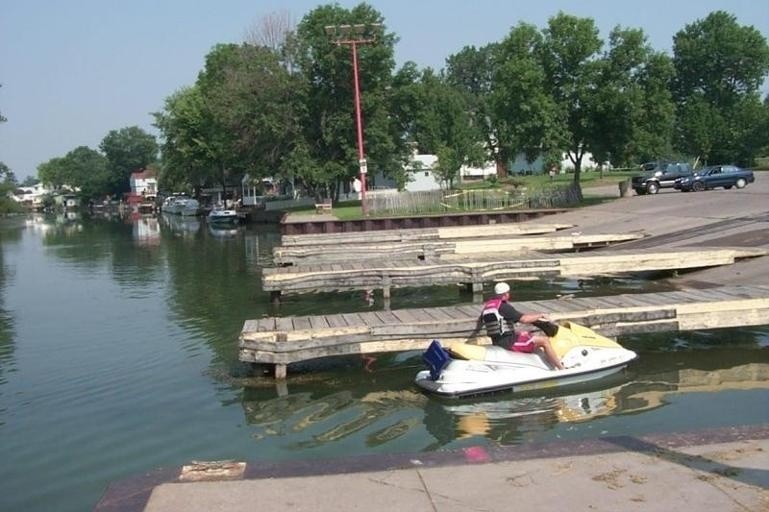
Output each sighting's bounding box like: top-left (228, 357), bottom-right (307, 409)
top-left (465, 282), bottom-right (566, 370)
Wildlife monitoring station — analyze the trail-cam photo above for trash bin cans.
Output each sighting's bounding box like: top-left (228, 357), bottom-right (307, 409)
top-left (619, 178), bottom-right (633, 198)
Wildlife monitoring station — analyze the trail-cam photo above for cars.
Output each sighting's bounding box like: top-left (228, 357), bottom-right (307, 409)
top-left (626, 158), bottom-right (756, 196)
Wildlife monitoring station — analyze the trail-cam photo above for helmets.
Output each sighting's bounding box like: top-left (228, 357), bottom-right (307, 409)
top-left (493, 281), bottom-right (511, 295)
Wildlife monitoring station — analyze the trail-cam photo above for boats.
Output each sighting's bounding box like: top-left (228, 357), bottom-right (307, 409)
top-left (422, 382), bottom-right (669, 446)
top-left (205, 198), bottom-right (245, 226)
top-left (408, 315), bottom-right (641, 399)
top-left (160, 192), bottom-right (204, 218)
top-left (206, 223), bottom-right (240, 238)
